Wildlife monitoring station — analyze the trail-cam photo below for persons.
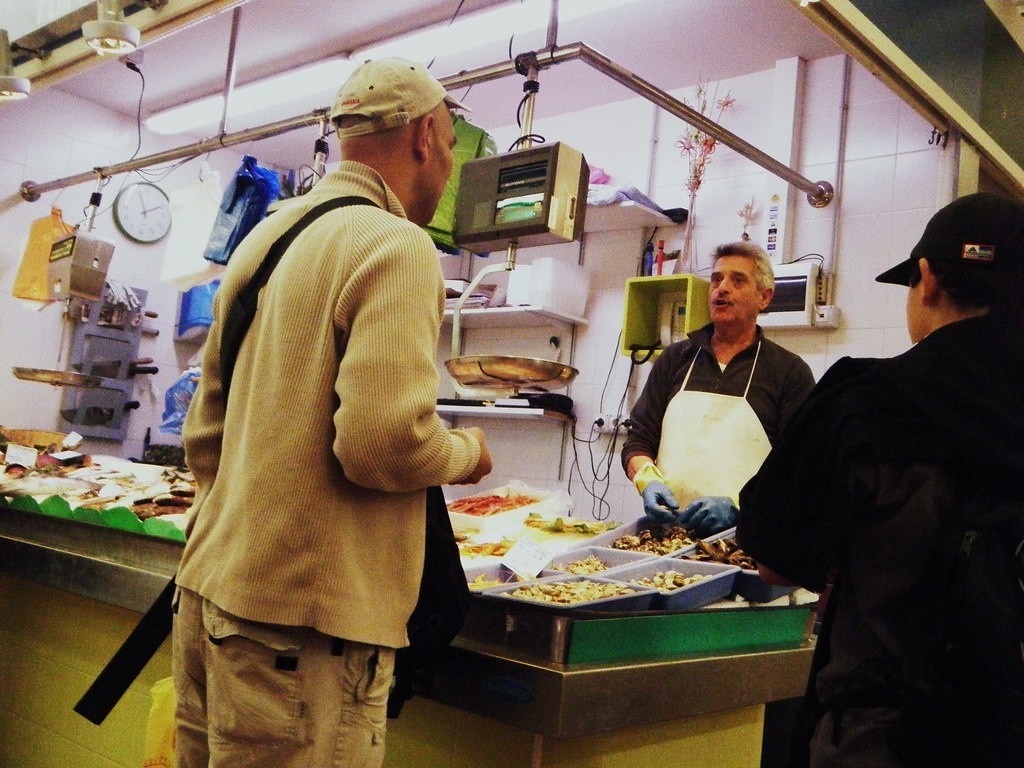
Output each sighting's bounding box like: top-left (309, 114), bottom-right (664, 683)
top-left (728, 187), bottom-right (1024, 768)
top-left (617, 234), bottom-right (823, 541)
top-left (146, 51), bottom-right (496, 768)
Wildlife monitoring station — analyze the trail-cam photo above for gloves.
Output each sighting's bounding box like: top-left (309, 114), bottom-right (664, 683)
top-left (680, 495), bottom-right (741, 537)
top-left (633, 463), bottom-right (677, 523)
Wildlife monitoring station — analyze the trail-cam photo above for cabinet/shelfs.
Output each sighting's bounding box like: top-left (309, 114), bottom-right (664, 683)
top-left (432, 288), bottom-right (590, 425)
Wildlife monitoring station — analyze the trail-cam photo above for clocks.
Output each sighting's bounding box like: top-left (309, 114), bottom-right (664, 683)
top-left (100, 176), bottom-right (179, 249)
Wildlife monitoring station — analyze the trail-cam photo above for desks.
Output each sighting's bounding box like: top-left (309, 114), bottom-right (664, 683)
top-left (0, 430), bottom-right (829, 768)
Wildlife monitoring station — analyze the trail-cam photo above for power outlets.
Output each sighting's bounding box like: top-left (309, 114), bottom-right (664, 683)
top-left (118, 49), bottom-right (144, 67)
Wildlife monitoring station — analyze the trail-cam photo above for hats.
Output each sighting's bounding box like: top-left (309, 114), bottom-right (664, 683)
top-left (333, 61), bottom-right (472, 141)
top-left (876, 191), bottom-right (1023, 287)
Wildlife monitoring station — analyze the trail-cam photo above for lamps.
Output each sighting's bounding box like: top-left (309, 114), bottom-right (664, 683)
top-left (0, 29), bottom-right (48, 100)
top-left (81, 0), bottom-right (169, 56)
